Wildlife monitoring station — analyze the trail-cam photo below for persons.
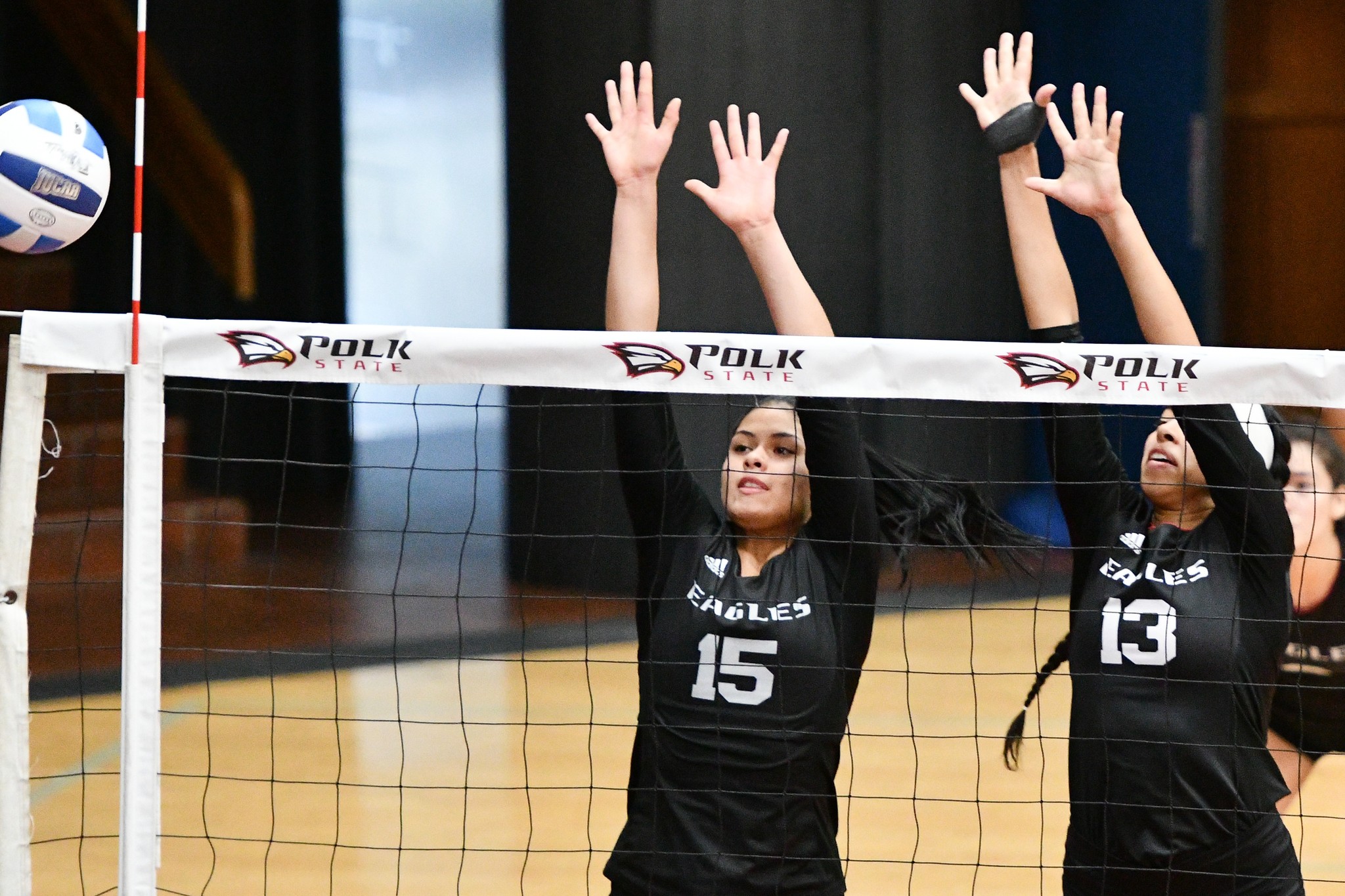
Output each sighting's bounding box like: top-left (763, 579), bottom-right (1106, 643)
top-left (957, 31), bottom-right (1345, 896)
top-left (586, 57), bottom-right (1051, 896)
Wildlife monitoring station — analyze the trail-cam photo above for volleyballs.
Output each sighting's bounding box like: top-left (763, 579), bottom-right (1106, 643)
top-left (0, 99), bottom-right (110, 255)
